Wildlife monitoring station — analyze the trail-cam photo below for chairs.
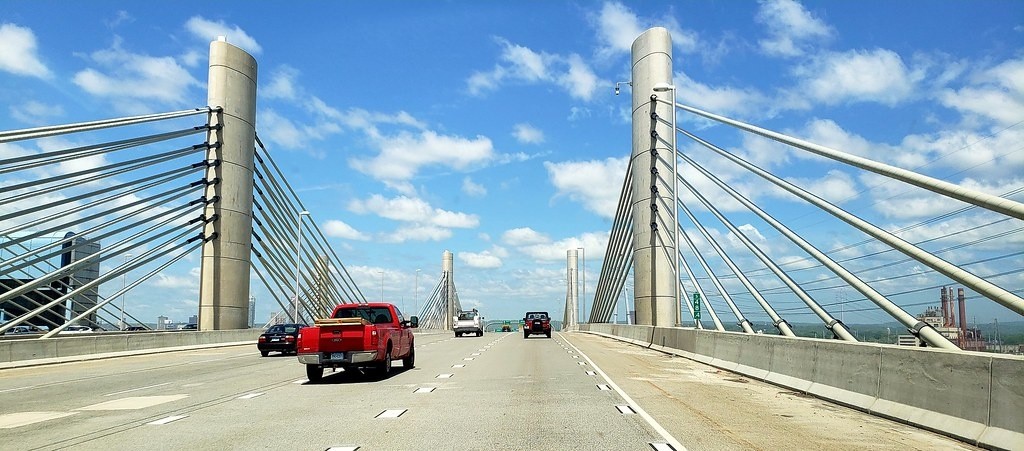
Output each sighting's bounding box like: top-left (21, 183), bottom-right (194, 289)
top-left (375, 314), bottom-right (388, 322)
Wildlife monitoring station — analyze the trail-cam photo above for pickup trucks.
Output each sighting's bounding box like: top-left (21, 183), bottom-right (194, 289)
top-left (453, 308), bottom-right (485, 337)
top-left (297, 303), bottom-right (418, 381)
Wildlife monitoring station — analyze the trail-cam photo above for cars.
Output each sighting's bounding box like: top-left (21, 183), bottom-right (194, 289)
top-left (123, 327), bottom-right (147, 331)
top-left (257, 324), bottom-right (310, 356)
top-left (182, 323), bottom-right (197, 329)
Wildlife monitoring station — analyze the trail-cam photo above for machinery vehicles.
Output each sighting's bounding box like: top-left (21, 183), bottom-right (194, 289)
top-left (502, 320), bottom-right (512, 332)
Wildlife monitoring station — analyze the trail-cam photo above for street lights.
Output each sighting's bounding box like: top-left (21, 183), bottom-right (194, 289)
top-left (416, 268), bottom-right (421, 316)
top-left (295, 211), bottom-right (310, 324)
top-left (653, 82), bottom-right (683, 327)
top-left (578, 246), bottom-right (587, 324)
top-left (377, 271), bottom-right (384, 302)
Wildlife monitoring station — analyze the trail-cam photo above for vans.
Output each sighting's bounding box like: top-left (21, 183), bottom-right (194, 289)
top-left (5, 326), bottom-right (49, 335)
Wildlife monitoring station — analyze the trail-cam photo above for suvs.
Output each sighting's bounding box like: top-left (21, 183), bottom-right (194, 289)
top-left (58, 326), bottom-right (93, 333)
top-left (523, 312), bottom-right (551, 338)
